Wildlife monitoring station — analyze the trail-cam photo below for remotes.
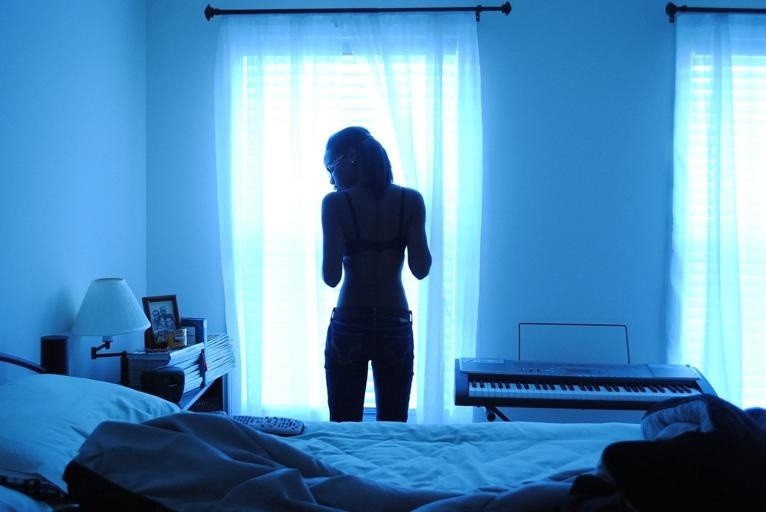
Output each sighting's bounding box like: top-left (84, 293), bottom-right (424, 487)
top-left (228, 414), bottom-right (304, 436)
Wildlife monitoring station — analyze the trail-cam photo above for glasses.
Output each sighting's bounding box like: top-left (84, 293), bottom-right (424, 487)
top-left (327, 152), bottom-right (348, 178)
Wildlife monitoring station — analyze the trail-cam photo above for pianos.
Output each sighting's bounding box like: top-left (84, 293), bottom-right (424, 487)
top-left (454, 357), bottom-right (717, 411)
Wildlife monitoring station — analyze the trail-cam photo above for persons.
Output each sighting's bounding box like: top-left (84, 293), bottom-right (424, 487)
top-left (151, 306), bottom-right (175, 342)
top-left (321, 128), bottom-right (431, 421)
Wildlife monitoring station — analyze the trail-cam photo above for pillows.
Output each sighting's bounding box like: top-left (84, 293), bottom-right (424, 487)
top-left (0, 374), bottom-right (181, 496)
top-left (0, 484), bottom-right (53, 512)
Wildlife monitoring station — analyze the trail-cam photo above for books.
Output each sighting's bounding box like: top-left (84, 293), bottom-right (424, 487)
top-left (126, 334), bottom-right (237, 394)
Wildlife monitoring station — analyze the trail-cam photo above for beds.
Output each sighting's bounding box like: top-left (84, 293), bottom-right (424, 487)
top-left (0, 335), bottom-right (765, 512)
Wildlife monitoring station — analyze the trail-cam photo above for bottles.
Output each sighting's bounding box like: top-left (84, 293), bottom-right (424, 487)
top-left (168, 329), bottom-right (188, 348)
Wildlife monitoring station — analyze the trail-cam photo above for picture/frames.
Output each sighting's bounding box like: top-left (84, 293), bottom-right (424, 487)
top-left (141, 294), bottom-right (181, 350)
top-left (181, 316), bottom-right (205, 388)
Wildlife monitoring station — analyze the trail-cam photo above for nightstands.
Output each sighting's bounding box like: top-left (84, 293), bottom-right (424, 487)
top-left (177, 373), bottom-right (228, 415)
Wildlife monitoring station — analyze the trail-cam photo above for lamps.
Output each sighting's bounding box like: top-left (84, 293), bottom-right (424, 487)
top-left (71, 277), bottom-right (152, 389)
